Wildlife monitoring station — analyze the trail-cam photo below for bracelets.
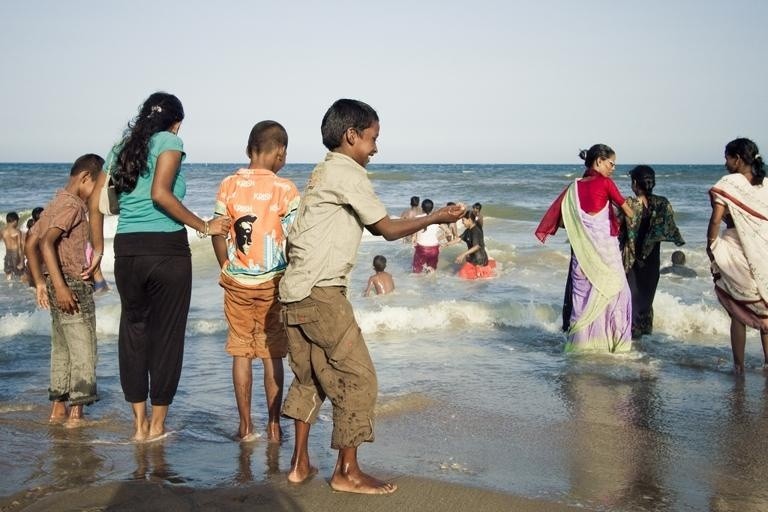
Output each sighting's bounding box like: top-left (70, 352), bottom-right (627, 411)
top-left (92, 248), bottom-right (104, 257)
top-left (706, 238), bottom-right (716, 245)
top-left (196, 216), bottom-right (212, 240)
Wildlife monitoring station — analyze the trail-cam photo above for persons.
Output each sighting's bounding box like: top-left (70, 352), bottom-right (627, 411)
top-left (23, 151), bottom-right (108, 431)
top-left (74, 88), bottom-right (232, 443)
top-left (614, 164), bottom-right (685, 341)
top-left (32, 207), bottom-right (43, 221)
top-left (706, 138), bottom-right (768, 376)
top-left (658, 250), bottom-right (697, 280)
top-left (1, 211), bottom-right (26, 284)
top-left (532, 144), bottom-right (636, 356)
top-left (132, 439), bottom-right (179, 485)
top-left (362, 255), bottom-right (396, 297)
top-left (401, 195), bottom-right (498, 282)
top-left (47, 424), bottom-right (101, 490)
top-left (21, 218), bottom-right (35, 283)
top-left (272, 98), bottom-right (463, 498)
top-left (233, 439), bottom-right (280, 487)
top-left (206, 119), bottom-right (299, 444)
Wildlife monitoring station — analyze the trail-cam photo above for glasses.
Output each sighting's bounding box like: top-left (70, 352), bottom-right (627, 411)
top-left (603, 157), bottom-right (615, 169)
